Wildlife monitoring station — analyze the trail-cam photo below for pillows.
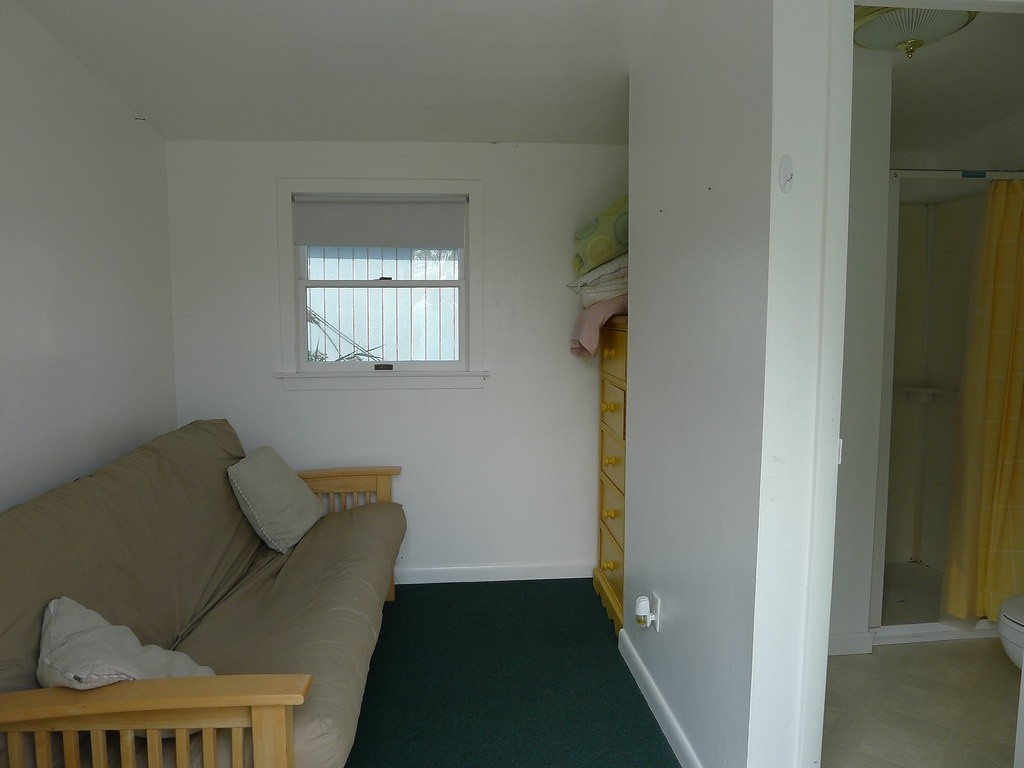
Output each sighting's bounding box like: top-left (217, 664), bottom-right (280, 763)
top-left (229, 445), bottom-right (327, 553)
top-left (36, 597), bottom-right (218, 738)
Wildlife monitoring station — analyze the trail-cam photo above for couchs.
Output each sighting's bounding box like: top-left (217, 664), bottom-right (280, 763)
top-left (0, 418), bottom-right (406, 768)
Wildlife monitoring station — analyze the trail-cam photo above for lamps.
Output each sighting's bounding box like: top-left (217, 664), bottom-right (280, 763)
top-left (853, 8), bottom-right (981, 59)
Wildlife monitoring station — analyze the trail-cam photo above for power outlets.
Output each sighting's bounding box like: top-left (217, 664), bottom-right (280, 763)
top-left (648, 590), bottom-right (661, 634)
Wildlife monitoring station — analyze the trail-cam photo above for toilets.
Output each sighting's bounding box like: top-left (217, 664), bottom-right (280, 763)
top-left (996, 593), bottom-right (1024, 671)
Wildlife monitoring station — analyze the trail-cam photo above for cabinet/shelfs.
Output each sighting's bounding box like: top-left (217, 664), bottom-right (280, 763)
top-left (593, 314), bottom-right (630, 638)
